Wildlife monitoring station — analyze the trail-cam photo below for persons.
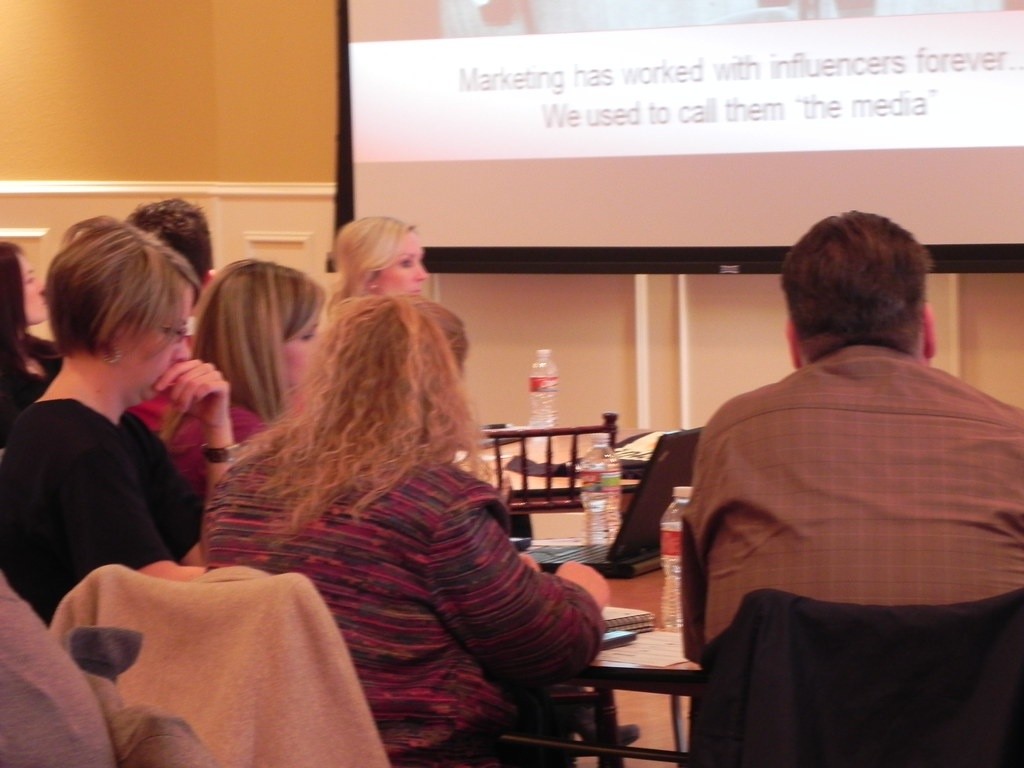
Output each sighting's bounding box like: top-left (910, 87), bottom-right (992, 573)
top-left (198, 292), bottom-right (638, 768)
top-left (324, 215), bottom-right (430, 316)
top-left (680, 211), bottom-right (1023, 670)
top-left (1, 242), bottom-right (62, 454)
top-left (160, 258), bottom-right (326, 504)
top-left (1, 215), bottom-right (208, 628)
top-left (121, 198), bottom-right (216, 366)
top-left (0, 560), bottom-right (393, 767)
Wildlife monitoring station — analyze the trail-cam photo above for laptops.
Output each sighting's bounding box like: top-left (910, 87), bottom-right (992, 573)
top-left (526, 427), bottom-right (701, 579)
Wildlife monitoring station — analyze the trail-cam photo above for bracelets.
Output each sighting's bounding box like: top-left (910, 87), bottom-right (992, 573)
top-left (201, 443), bottom-right (241, 463)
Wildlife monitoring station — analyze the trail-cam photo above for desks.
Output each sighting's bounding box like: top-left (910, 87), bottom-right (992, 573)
top-left (508, 533), bottom-right (704, 768)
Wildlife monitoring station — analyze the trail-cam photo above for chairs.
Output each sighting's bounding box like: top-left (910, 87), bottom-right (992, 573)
top-left (676, 583), bottom-right (1024, 766)
top-left (44, 563), bottom-right (349, 768)
top-left (466, 412), bottom-right (631, 514)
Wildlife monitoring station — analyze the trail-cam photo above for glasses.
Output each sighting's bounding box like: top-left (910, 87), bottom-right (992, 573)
top-left (154, 322), bottom-right (189, 342)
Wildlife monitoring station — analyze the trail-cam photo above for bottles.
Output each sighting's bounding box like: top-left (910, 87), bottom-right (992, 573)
top-left (658, 486), bottom-right (692, 627)
top-left (578, 431), bottom-right (622, 543)
top-left (530, 350), bottom-right (558, 429)
